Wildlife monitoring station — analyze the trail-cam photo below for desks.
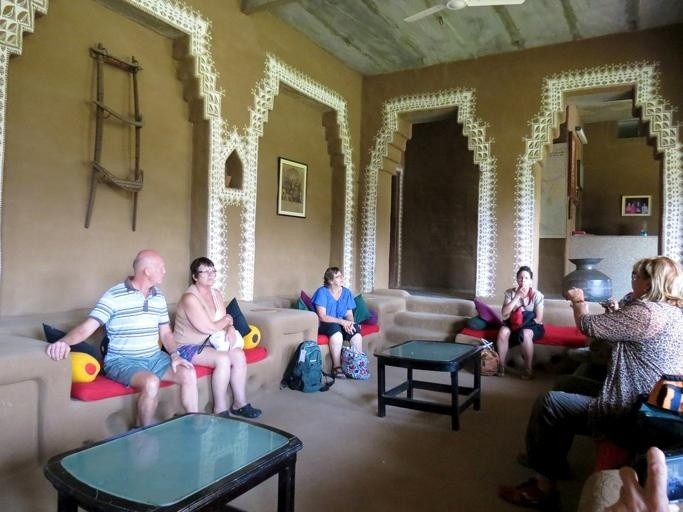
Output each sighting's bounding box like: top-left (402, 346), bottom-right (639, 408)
top-left (44, 412), bottom-right (303, 512)
top-left (374, 339), bottom-right (484, 432)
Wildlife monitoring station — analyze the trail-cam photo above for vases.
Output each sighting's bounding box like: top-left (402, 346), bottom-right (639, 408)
top-left (562, 257), bottom-right (612, 301)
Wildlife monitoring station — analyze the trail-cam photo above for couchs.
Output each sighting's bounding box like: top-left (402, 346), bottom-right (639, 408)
top-left (254, 288), bottom-right (377, 376)
top-left (590, 401), bottom-right (683, 512)
top-left (364, 288), bottom-right (478, 357)
top-left (0, 301), bottom-right (319, 476)
top-left (457, 297), bottom-right (606, 373)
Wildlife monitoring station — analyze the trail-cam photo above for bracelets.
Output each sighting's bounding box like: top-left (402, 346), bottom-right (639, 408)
top-left (570, 300), bottom-right (584, 307)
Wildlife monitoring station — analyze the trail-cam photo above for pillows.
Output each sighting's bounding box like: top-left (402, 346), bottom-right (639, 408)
top-left (68, 351), bottom-right (101, 383)
top-left (359, 308), bottom-right (378, 325)
top-left (300, 291), bottom-right (312, 308)
top-left (43, 323), bottom-right (99, 362)
top-left (352, 294), bottom-right (371, 323)
top-left (467, 316), bottom-right (489, 330)
top-left (473, 297), bottom-right (504, 327)
top-left (242, 324), bottom-right (261, 349)
top-left (225, 298), bottom-right (251, 337)
top-left (294, 297), bottom-right (309, 311)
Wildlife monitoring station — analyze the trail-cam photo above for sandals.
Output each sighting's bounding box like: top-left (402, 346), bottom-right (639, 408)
top-left (231, 402), bottom-right (262, 418)
top-left (331, 366), bottom-right (346, 379)
top-left (218, 410), bottom-right (230, 417)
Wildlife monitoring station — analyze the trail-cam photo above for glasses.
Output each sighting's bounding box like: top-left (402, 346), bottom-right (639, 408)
top-left (334, 274), bottom-right (344, 279)
top-left (199, 268), bottom-right (216, 274)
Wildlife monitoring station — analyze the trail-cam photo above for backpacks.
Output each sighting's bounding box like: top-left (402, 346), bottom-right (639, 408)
top-left (280, 339), bottom-right (335, 394)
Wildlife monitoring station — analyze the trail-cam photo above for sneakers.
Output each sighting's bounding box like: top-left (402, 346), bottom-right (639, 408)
top-left (496, 366), bottom-right (505, 377)
top-left (497, 478), bottom-right (549, 507)
top-left (520, 367), bottom-right (532, 379)
top-left (520, 450), bottom-right (531, 466)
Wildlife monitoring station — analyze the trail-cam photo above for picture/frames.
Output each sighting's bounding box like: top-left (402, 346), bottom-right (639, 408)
top-left (276, 155), bottom-right (309, 219)
top-left (621, 194), bottom-right (652, 216)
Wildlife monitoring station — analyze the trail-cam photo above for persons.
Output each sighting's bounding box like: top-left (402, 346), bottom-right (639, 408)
top-left (46, 249), bottom-right (199, 429)
top-left (603, 446), bottom-right (670, 512)
top-left (587, 257), bottom-right (651, 372)
top-left (496, 256), bottom-right (683, 507)
top-left (309, 266), bottom-right (362, 379)
top-left (173, 256), bottom-right (262, 419)
top-left (496, 265), bottom-right (544, 380)
top-left (626, 201), bottom-right (648, 214)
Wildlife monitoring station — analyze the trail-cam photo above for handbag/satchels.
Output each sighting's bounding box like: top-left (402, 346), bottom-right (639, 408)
top-left (342, 344), bottom-right (369, 379)
top-left (478, 338), bottom-right (501, 376)
